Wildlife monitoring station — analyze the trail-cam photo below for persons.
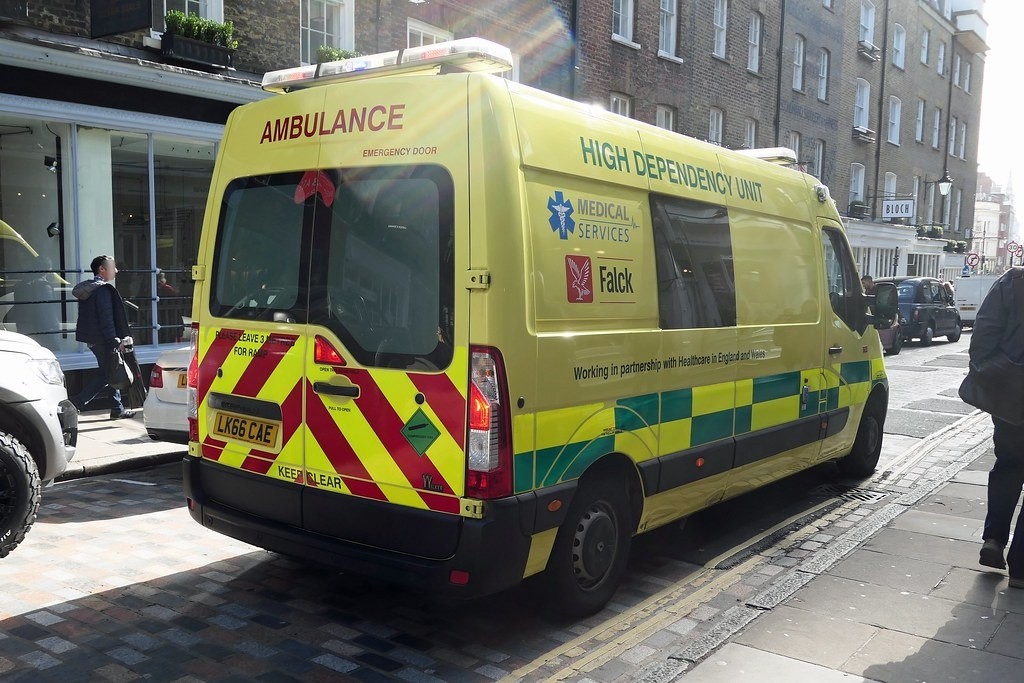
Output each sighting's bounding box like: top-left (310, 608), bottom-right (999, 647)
top-left (65, 256), bottom-right (138, 421)
top-left (958, 264), bottom-right (1024, 593)
top-left (15, 258), bottom-right (173, 353)
top-left (859, 275), bottom-right (872, 288)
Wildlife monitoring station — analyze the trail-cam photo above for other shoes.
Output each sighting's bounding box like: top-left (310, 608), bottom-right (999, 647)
top-left (109, 411), bottom-right (137, 419)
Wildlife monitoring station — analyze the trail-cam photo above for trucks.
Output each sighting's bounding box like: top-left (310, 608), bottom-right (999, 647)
top-left (954, 274), bottom-right (1002, 327)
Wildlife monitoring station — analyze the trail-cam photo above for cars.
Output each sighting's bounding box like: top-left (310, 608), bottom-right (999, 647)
top-left (0, 330), bottom-right (80, 562)
top-left (141, 346), bottom-right (198, 446)
top-left (2, 287), bottom-right (192, 346)
top-left (875, 275), bottom-right (963, 346)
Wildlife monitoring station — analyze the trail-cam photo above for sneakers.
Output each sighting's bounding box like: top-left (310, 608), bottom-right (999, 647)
top-left (980, 539), bottom-right (1006, 569)
top-left (1008, 577), bottom-right (1023, 588)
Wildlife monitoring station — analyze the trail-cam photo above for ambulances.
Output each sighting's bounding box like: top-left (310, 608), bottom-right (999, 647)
top-left (1, 220), bottom-right (71, 285)
top-left (177, 37), bottom-right (899, 626)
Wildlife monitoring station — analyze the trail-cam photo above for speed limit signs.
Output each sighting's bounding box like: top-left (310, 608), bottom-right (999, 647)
top-left (1007, 242), bottom-right (1018, 253)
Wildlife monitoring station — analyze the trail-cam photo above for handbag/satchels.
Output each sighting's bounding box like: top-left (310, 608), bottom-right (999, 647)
top-left (105, 348), bottom-right (134, 390)
